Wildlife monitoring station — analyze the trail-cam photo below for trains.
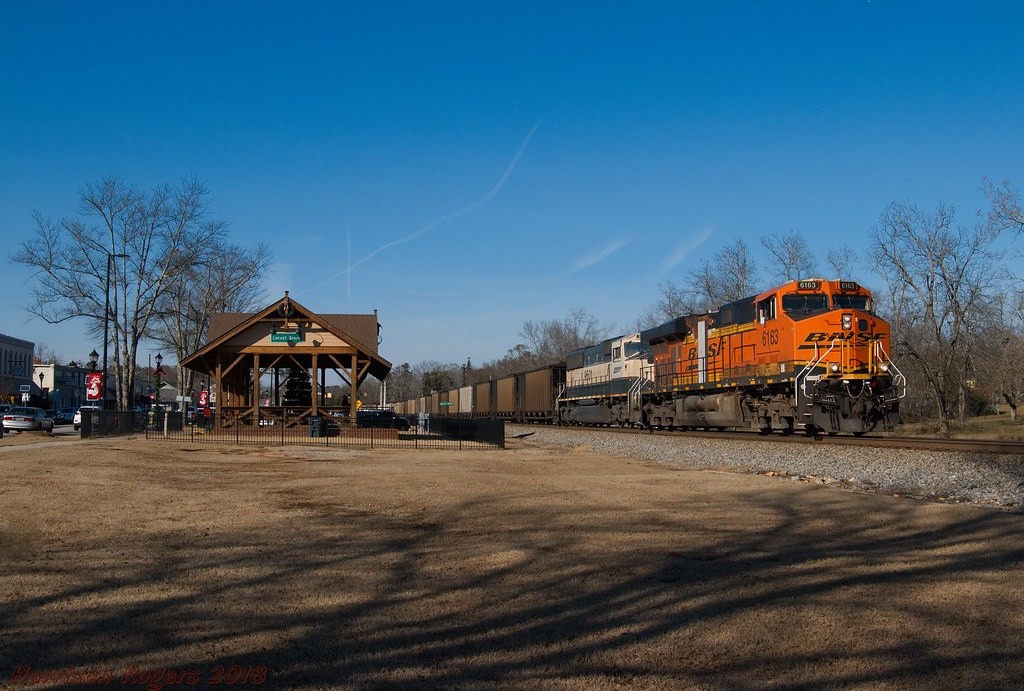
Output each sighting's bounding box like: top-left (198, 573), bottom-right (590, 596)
top-left (356, 277), bottom-right (907, 438)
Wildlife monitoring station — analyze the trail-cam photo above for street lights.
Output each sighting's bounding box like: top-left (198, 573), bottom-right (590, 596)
top-left (102, 252), bottom-right (131, 410)
top-left (154, 353), bottom-right (164, 427)
top-left (39, 372), bottom-right (44, 397)
top-left (200, 378), bottom-right (205, 391)
top-left (88, 349), bottom-right (100, 372)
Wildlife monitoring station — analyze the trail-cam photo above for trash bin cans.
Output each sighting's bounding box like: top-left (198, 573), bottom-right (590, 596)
top-left (308, 416), bottom-right (323, 437)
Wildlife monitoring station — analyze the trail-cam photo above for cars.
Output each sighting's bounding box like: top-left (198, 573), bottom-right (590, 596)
top-left (59, 406), bottom-right (79, 423)
top-left (73, 406), bottom-right (107, 431)
top-left (357, 409), bottom-right (411, 431)
top-left (0, 404), bottom-right (55, 438)
top-left (178, 406), bottom-right (200, 421)
top-left (44, 409), bottom-right (64, 425)
